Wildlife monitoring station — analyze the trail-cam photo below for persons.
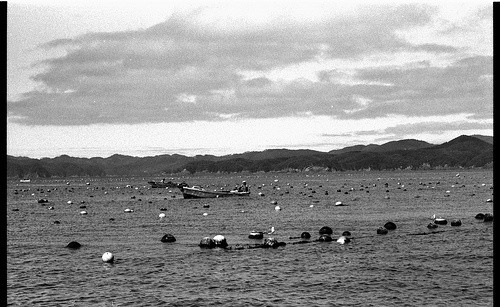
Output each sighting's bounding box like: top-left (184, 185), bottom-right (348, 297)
top-left (238, 180), bottom-right (247, 192)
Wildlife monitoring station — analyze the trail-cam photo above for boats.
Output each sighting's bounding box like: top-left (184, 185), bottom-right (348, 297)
top-left (148, 179), bottom-right (189, 188)
top-left (177, 184), bottom-right (250, 199)
